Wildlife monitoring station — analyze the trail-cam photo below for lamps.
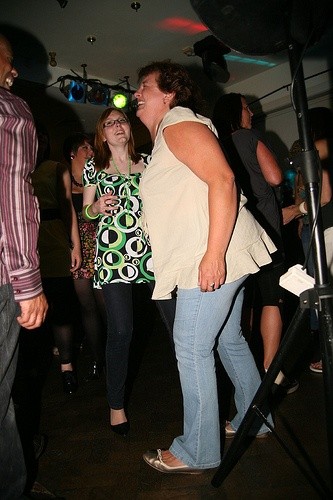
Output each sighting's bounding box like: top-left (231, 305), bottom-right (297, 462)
top-left (61, 78), bottom-right (134, 115)
top-left (192, 35), bottom-right (230, 83)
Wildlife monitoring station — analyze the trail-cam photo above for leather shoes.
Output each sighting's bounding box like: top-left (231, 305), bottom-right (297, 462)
top-left (141, 450), bottom-right (205, 475)
top-left (224, 422), bottom-right (271, 440)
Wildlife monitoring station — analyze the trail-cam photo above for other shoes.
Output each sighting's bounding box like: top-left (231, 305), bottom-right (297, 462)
top-left (87, 363), bottom-right (101, 392)
top-left (272, 381), bottom-right (299, 394)
top-left (309, 363), bottom-right (323, 373)
top-left (61, 369), bottom-right (78, 399)
top-left (103, 399), bottom-right (130, 436)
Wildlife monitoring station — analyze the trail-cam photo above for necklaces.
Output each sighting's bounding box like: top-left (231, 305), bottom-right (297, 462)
top-left (70, 175), bottom-right (82, 188)
top-left (110, 155), bottom-right (132, 218)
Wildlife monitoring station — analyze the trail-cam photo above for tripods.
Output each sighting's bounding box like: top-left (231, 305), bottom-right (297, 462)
top-left (212, 58), bottom-right (333, 500)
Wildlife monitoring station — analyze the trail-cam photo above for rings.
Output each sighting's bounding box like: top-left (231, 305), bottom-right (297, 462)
top-left (207, 282), bottom-right (215, 287)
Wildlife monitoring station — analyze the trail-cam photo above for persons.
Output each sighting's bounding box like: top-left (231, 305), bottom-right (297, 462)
top-left (134, 58), bottom-right (273, 473)
top-left (50, 91), bottom-right (333, 440)
top-left (0, 39), bottom-right (49, 500)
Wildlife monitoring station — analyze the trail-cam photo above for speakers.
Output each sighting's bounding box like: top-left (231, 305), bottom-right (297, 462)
top-left (191, 0), bottom-right (333, 59)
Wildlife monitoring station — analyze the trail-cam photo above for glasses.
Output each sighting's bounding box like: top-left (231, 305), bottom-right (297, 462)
top-left (103, 117), bottom-right (128, 128)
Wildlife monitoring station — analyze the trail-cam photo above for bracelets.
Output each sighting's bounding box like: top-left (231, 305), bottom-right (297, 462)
top-left (299, 201), bottom-right (307, 214)
